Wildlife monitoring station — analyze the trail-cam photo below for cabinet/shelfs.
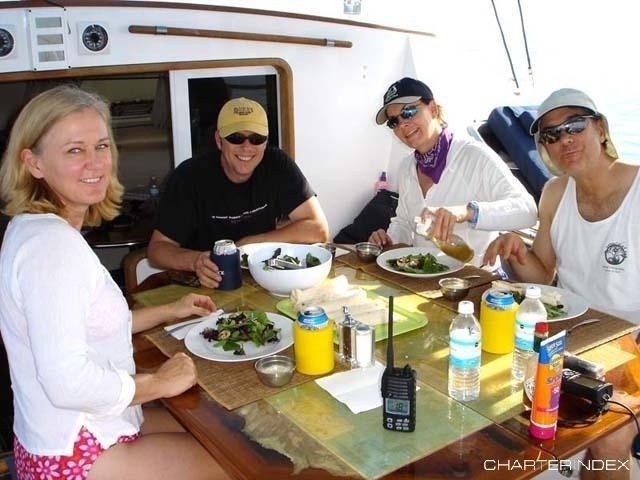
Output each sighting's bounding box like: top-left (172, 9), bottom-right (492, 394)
top-left (447, 301), bottom-right (484, 404)
top-left (337, 305), bottom-right (375, 367)
top-left (414, 219), bottom-right (477, 263)
top-left (511, 283), bottom-right (550, 413)
top-left (149, 175), bottom-right (160, 198)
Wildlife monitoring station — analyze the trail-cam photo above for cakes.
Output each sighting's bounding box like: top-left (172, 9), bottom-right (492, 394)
top-left (207, 236), bottom-right (240, 292)
top-left (479, 285), bottom-right (517, 355)
top-left (295, 306), bottom-right (333, 375)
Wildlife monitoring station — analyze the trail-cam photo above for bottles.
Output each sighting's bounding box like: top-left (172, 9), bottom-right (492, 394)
top-left (216, 96), bottom-right (270, 140)
top-left (374, 76), bottom-right (434, 125)
top-left (527, 88), bottom-right (600, 137)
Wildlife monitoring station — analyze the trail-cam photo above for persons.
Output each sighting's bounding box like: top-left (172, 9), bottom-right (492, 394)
top-left (484, 89), bottom-right (639, 479)
top-left (369, 78), bottom-right (537, 277)
top-left (1, 85), bottom-right (228, 480)
top-left (147, 96), bottom-right (329, 289)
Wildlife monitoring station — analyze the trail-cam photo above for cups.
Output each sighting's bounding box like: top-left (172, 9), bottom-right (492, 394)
top-left (385, 103), bottom-right (417, 128)
top-left (537, 112), bottom-right (597, 145)
top-left (225, 132), bottom-right (267, 147)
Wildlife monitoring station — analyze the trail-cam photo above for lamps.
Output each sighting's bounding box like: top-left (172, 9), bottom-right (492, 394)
top-left (124, 242), bottom-right (171, 293)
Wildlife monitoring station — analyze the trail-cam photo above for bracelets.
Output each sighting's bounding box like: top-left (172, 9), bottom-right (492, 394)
top-left (439, 278), bottom-right (472, 300)
top-left (247, 245), bottom-right (332, 297)
top-left (313, 243), bottom-right (336, 261)
top-left (355, 242), bottom-right (383, 260)
top-left (254, 354), bottom-right (295, 387)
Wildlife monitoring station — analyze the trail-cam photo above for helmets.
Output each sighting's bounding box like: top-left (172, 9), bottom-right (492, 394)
top-left (481, 283), bottom-right (591, 325)
top-left (376, 246), bottom-right (468, 277)
top-left (184, 310), bottom-right (296, 362)
top-left (234, 242), bottom-right (294, 271)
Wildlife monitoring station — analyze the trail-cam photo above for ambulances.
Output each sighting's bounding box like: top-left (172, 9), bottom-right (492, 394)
top-left (289, 272), bottom-right (395, 330)
